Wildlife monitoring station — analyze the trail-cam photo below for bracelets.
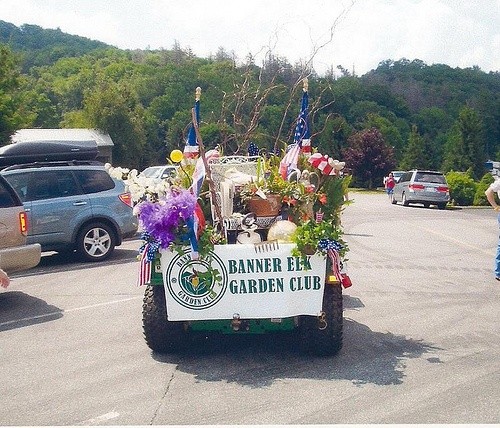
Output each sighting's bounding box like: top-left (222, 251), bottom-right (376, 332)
top-left (494, 205), bottom-right (499, 211)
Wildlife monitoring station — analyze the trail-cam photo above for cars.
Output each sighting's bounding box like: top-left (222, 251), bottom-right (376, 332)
top-left (122, 164), bottom-right (179, 190)
top-left (389, 169), bottom-right (450, 209)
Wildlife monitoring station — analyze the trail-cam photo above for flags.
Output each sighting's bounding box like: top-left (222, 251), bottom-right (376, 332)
top-left (179, 98), bottom-right (211, 260)
top-left (278, 89), bottom-right (311, 179)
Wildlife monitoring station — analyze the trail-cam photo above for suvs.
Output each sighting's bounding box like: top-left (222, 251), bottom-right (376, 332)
top-left (0, 139), bottom-right (139, 274)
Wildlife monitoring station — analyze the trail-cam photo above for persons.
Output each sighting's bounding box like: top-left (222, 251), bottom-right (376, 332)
top-left (385, 173), bottom-right (395, 199)
top-left (485, 179), bottom-right (500, 281)
top-left (339, 171), bottom-right (349, 200)
